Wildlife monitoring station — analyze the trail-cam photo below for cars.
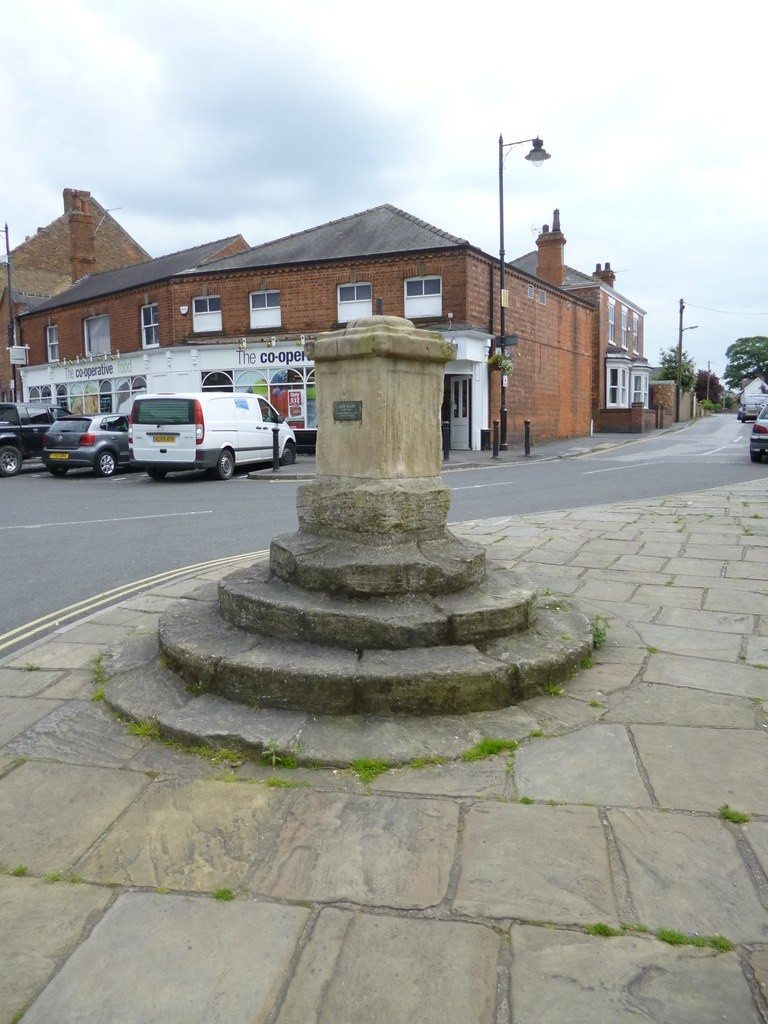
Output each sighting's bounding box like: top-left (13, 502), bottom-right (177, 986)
top-left (41, 411), bottom-right (142, 477)
top-left (739, 395), bottom-right (768, 423)
top-left (749, 403), bottom-right (768, 463)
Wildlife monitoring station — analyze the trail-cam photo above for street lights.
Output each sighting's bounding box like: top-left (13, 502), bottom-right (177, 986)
top-left (676, 325), bottom-right (699, 423)
top-left (495, 133), bottom-right (554, 451)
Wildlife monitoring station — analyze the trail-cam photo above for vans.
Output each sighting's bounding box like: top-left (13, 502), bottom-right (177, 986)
top-left (128, 391), bottom-right (297, 481)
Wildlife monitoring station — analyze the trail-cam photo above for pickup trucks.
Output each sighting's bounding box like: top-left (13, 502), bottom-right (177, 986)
top-left (0, 402), bottom-right (76, 477)
